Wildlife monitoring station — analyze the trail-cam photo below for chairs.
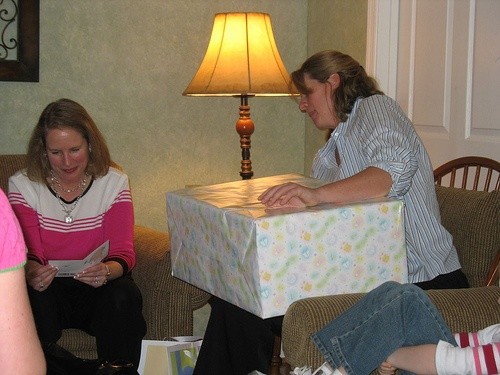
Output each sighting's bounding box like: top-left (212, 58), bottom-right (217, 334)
top-left (273, 157), bottom-right (500, 375)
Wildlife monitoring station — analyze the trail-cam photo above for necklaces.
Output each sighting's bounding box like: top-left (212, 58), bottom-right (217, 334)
top-left (51, 170), bottom-right (91, 224)
top-left (51, 169), bottom-right (84, 194)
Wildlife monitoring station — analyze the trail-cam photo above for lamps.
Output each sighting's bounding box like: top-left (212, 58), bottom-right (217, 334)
top-left (183, 11), bottom-right (301, 180)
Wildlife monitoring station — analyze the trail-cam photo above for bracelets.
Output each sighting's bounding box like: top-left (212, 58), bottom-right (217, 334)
top-left (104, 265), bottom-right (110, 285)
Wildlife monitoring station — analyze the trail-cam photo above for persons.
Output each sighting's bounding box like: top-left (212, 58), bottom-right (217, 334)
top-left (1, 98), bottom-right (146, 375)
top-left (194, 51), bottom-right (500, 375)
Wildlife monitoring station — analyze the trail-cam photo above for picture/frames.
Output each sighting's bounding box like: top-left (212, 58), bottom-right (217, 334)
top-left (0, 0), bottom-right (39, 82)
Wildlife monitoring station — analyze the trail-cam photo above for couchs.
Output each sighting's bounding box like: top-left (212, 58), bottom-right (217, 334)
top-left (0, 154), bottom-right (212, 360)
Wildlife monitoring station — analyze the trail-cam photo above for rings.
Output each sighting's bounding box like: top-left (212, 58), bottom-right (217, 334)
top-left (96, 281), bottom-right (99, 284)
top-left (95, 277), bottom-right (99, 281)
top-left (39, 282), bottom-right (44, 288)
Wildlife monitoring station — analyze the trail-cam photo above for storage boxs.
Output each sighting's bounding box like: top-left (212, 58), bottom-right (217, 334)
top-left (167, 173), bottom-right (407, 319)
